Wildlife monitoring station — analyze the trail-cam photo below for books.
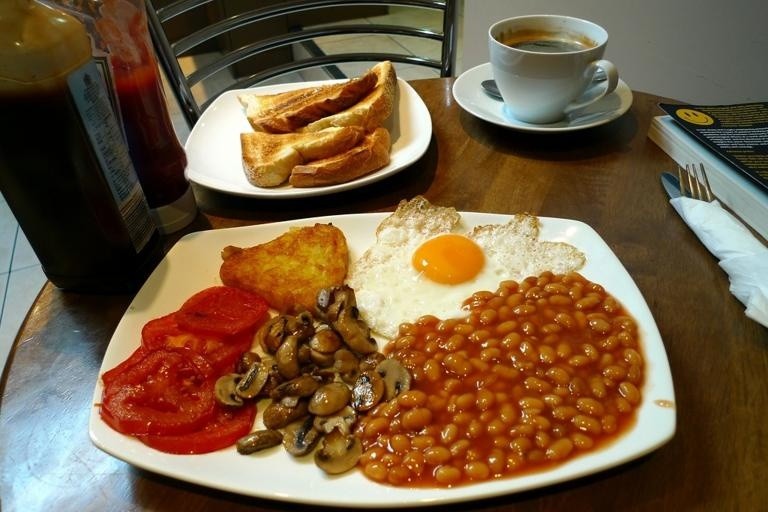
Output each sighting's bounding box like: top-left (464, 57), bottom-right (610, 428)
top-left (646, 99), bottom-right (766, 245)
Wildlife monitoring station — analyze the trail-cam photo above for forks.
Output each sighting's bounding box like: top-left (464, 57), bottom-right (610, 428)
top-left (677, 162), bottom-right (714, 203)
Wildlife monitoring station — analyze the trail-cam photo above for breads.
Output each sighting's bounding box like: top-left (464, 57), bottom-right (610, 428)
top-left (237, 60), bottom-right (397, 188)
top-left (221, 222), bottom-right (349, 313)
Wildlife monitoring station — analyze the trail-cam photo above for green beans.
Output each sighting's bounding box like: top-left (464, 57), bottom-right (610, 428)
top-left (218, 272), bottom-right (641, 484)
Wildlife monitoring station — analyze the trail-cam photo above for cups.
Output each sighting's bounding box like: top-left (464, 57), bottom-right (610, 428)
top-left (485, 11), bottom-right (620, 125)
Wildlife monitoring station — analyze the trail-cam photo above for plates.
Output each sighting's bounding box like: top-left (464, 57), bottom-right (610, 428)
top-left (86, 205), bottom-right (681, 510)
top-left (182, 72), bottom-right (434, 202)
top-left (450, 60), bottom-right (637, 135)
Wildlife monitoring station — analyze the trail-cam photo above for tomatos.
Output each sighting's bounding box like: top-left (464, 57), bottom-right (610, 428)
top-left (100, 285), bottom-right (271, 455)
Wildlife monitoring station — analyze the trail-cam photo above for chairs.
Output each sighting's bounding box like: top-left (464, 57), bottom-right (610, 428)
top-left (147, 0), bottom-right (462, 129)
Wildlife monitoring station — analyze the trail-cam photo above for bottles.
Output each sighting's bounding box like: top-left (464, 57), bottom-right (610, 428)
top-left (0, 0), bottom-right (200, 294)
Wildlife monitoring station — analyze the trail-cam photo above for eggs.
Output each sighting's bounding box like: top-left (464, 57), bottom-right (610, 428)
top-left (343, 195), bottom-right (583, 340)
